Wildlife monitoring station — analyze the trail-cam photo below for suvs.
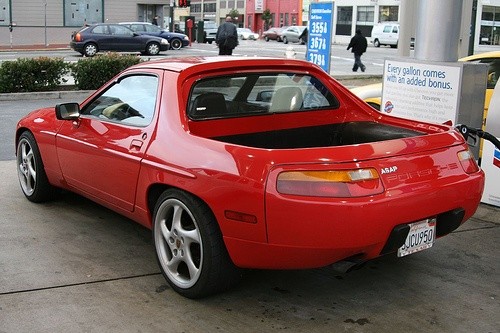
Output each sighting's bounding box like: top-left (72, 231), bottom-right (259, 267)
top-left (202, 21), bottom-right (219, 44)
top-left (121, 22), bottom-right (190, 50)
top-left (71, 23), bottom-right (170, 57)
top-left (174, 23), bottom-right (185, 33)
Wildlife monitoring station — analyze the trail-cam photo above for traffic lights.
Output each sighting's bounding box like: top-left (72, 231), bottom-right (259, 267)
top-left (178, 0), bottom-right (191, 7)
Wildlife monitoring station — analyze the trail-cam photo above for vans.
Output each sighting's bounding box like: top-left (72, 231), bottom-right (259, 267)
top-left (370, 24), bottom-right (414, 48)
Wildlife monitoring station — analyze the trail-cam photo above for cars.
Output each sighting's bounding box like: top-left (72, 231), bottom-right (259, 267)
top-left (237, 28), bottom-right (259, 41)
top-left (349, 49), bottom-right (500, 165)
top-left (262, 27), bottom-right (284, 42)
top-left (14, 55), bottom-right (486, 299)
top-left (281, 26), bottom-right (307, 45)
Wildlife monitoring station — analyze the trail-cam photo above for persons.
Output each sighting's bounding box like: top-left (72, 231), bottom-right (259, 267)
top-left (298, 27), bottom-right (308, 58)
top-left (347, 28), bottom-right (368, 73)
top-left (215, 16), bottom-right (239, 56)
top-left (152, 14), bottom-right (159, 26)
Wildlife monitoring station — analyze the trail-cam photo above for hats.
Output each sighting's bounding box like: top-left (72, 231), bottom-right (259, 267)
top-left (226, 16), bottom-right (233, 22)
top-left (355, 28), bottom-right (361, 34)
